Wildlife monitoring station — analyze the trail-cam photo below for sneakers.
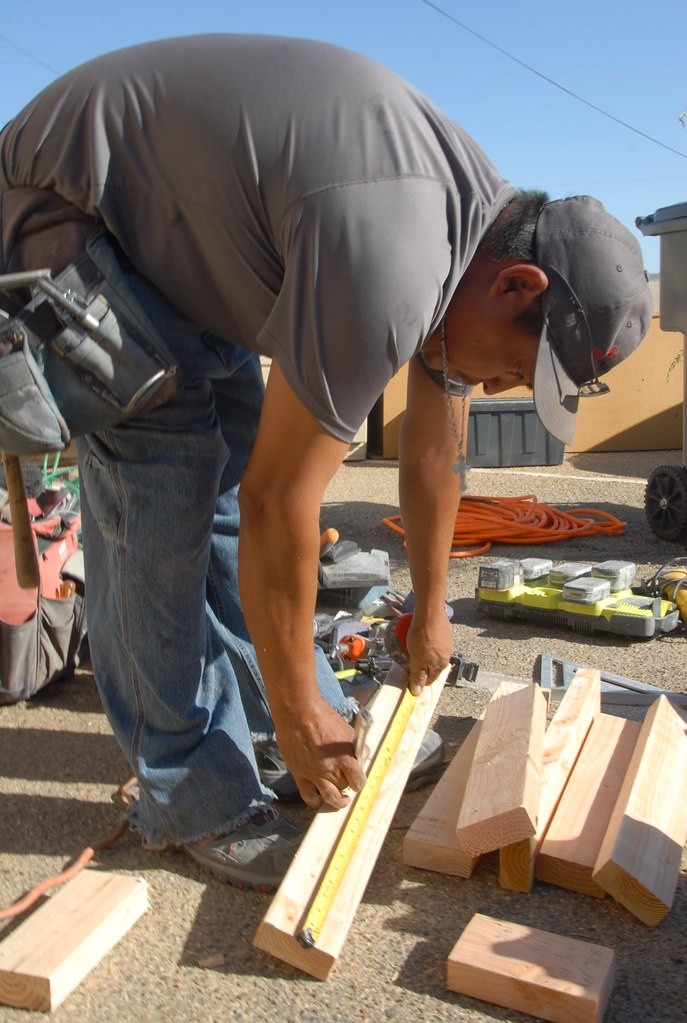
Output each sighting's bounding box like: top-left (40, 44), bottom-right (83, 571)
top-left (180, 802), bottom-right (305, 894)
top-left (252, 728), bottom-right (445, 794)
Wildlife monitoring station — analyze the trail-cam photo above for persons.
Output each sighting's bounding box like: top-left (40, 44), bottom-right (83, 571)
top-left (0, 33), bottom-right (653, 893)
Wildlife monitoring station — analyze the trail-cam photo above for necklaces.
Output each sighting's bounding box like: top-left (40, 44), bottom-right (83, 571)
top-left (440, 318), bottom-right (470, 492)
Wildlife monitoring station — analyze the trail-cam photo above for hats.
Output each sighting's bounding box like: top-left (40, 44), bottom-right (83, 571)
top-left (534, 194), bottom-right (652, 447)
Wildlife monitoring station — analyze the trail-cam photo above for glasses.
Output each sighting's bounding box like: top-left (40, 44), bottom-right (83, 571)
top-left (547, 263), bottom-right (610, 398)
